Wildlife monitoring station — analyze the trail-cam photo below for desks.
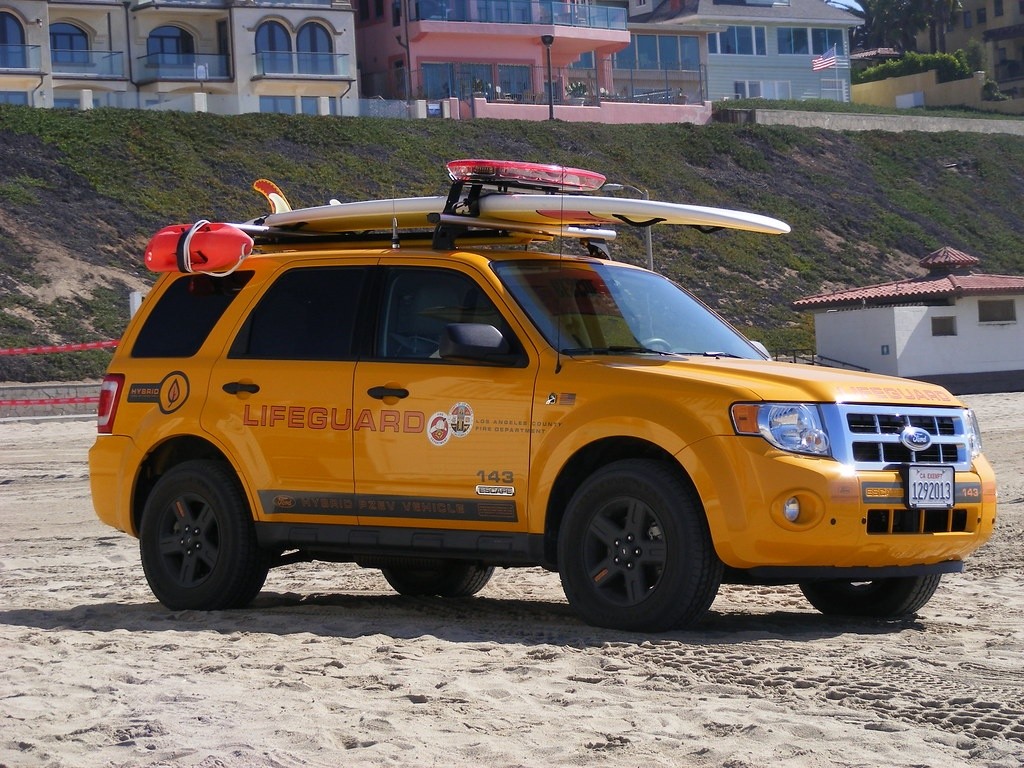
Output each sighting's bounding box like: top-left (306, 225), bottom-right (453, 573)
top-left (488, 99), bottom-right (514, 104)
top-left (543, 12), bottom-right (577, 27)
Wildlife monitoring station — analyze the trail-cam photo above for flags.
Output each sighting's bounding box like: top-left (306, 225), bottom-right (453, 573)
top-left (811, 47), bottom-right (835, 71)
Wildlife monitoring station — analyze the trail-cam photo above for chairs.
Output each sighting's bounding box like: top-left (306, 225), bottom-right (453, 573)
top-left (489, 86), bottom-right (548, 105)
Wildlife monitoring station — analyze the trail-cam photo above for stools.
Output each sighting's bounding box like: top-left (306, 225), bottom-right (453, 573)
top-left (577, 18), bottom-right (586, 27)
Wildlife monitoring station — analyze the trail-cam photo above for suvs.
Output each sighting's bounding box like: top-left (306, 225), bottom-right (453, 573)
top-left (85, 159), bottom-right (999, 634)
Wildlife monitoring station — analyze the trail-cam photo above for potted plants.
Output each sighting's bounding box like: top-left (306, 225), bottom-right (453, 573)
top-left (565, 81), bottom-right (588, 106)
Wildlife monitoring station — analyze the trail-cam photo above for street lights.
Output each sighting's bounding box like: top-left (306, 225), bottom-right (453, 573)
top-left (539, 33), bottom-right (554, 121)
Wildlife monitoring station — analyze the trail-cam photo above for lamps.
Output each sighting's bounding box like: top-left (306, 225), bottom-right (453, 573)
top-left (37, 18), bottom-right (42, 29)
